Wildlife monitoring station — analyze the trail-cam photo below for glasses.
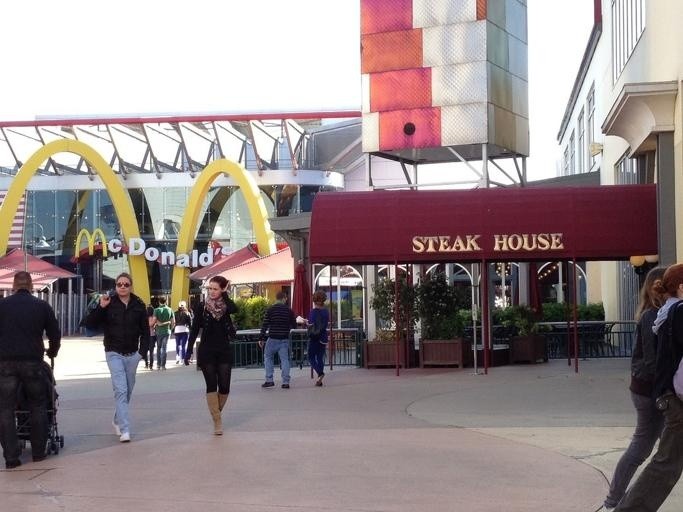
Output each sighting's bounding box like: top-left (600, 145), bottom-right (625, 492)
top-left (117, 282), bottom-right (131, 288)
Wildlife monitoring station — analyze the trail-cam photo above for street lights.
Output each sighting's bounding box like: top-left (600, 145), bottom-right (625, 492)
top-left (21, 220), bottom-right (50, 287)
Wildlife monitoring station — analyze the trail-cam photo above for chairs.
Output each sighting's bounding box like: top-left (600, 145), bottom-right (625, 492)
top-left (586, 322), bottom-right (616, 356)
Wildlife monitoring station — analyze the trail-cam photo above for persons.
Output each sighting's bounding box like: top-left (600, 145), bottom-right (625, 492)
top-left (602, 265), bottom-right (669, 512)
top-left (0, 271), bottom-right (62, 469)
top-left (184, 275), bottom-right (239, 435)
top-left (258, 291), bottom-right (297, 388)
top-left (86, 272), bottom-right (151, 442)
top-left (149, 295), bottom-right (176, 370)
top-left (303, 290), bottom-right (329, 386)
top-left (172, 301), bottom-right (192, 364)
top-left (614, 262), bottom-right (683, 511)
top-left (143, 306), bottom-right (171, 370)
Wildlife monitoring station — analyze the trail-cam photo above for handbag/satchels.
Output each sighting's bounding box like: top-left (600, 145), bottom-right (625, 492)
top-left (307, 307), bottom-right (323, 337)
top-left (79, 292), bottom-right (108, 333)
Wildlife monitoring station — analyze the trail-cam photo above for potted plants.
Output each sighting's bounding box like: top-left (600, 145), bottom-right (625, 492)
top-left (414, 272), bottom-right (472, 369)
top-left (364, 276), bottom-right (414, 369)
top-left (498, 305), bottom-right (548, 363)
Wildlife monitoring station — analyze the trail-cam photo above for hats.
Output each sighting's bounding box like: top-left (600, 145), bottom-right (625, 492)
top-left (179, 301), bottom-right (186, 308)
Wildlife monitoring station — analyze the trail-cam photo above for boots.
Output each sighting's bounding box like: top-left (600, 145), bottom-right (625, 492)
top-left (218, 393), bottom-right (229, 411)
top-left (204, 391), bottom-right (224, 435)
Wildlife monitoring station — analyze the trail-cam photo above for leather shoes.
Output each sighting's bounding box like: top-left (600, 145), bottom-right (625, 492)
top-left (5, 459), bottom-right (22, 468)
top-left (281, 383), bottom-right (289, 389)
top-left (262, 382), bottom-right (274, 388)
top-left (32, 452), bottom-right (47, 461)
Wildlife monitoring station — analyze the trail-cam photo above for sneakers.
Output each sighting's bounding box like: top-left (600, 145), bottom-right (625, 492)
top-left (175, 355), bottom-right (180, 364)
top-left (181, 358), bottom-right (185, 366)
top-left (118, 431), bottom-right (130, 442)
top-left (112, 418), bottom-right (120, 437)
top-left (146, 365), bottom-right (166, 371)
top-left (316, 373), bottom-right (324, 386)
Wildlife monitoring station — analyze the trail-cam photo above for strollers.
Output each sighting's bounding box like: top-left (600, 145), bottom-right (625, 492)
top-left (10, 342), bottom-right (70, 456)
